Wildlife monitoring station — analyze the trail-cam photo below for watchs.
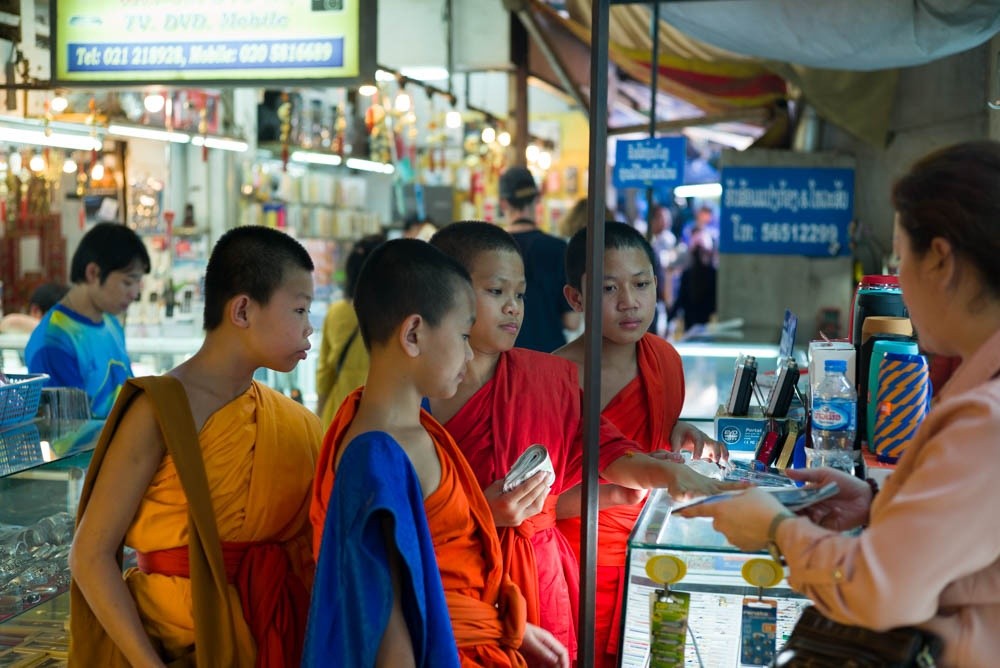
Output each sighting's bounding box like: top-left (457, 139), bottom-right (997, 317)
top-left (767, 513), bottom-right (796, 567)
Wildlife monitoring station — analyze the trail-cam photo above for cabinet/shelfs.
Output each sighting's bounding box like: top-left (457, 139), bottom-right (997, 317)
top-left (618, 457), bottom-right (816, 668)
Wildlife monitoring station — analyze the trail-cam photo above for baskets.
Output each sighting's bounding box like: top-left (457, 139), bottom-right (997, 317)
top-left (0, 373), bottom-right (50, 427)
top-left (0, 423), bottom-right (45, 475)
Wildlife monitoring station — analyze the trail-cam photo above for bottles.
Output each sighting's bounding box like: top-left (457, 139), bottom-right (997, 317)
top-left (859, 316), bottom-right (914, 440)
top-left (866, 340), bottom-right (920, 453)
top-left (874, 353), bottom-right (931, 466)
top-left (810, 360), bottom-right (857, 478)
top-left (848, 273), bottom-right (900, 343)
top-left (852, 289), bottom-right (908, 359)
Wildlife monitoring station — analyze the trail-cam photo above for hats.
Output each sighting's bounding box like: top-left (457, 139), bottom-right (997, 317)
top-left (500, 167), bottom-right (538, 199)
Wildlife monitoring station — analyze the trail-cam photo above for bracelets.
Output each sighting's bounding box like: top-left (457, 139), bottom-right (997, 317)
top-left (864, 478), bottom-right (880, 498)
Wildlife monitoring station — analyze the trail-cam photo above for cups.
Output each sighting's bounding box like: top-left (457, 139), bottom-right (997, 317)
top-left (793, 105), bottom-right (823, 153)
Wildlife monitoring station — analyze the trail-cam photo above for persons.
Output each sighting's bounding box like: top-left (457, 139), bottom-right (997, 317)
top-left (561, 201), bottom-right (665, 343)
top-left (430, 221), bottom-right (752, 668)
top-left (549, 221), bottom-right (729, 668)
top-left (682, 140), bottom-right (999, 668)
top-left (24, 221), bottom-right (152, 469)
top-left (315, 235), bottom-right (385, 434)
top-left (310, 238), bottom-right (571, 668)
top-left (497, 168), bottom-right (578, 355)
top-left (68, 225), bottom-right (326, 668)
top-left (647, 206), bottom-right (687, 322)
top-left (402, 214), bottom-right (439, 243)
top-left (0, 282), bottom-right (70, 334)
top-left (676, 205), bottom-right (718, 328)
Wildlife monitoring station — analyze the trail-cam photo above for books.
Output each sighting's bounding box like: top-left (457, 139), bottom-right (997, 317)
top-left (671, 481), bottom-right (840, 512)
